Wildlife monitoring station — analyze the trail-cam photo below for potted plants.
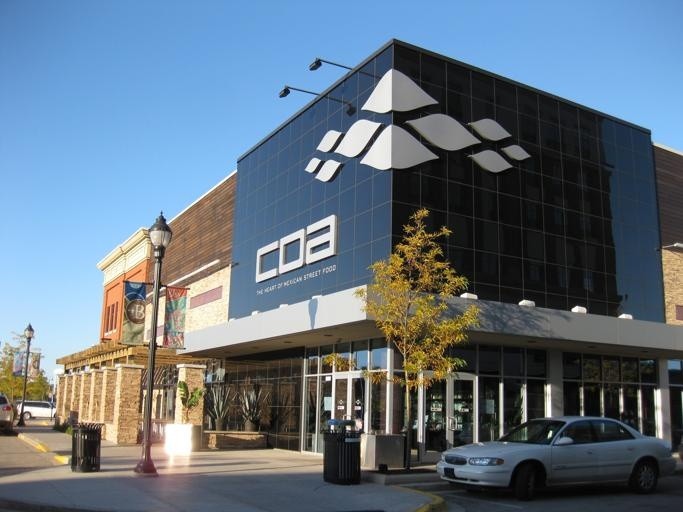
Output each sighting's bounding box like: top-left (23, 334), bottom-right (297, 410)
top-left (203, 386), bottom-right (271, 432)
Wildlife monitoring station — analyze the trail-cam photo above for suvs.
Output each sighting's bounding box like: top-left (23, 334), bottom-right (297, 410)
top-left (0, 395), bottom-right (57, 434)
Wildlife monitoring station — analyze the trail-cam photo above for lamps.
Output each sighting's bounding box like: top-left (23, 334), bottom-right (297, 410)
top-left (322, 96), bottom-right (356, 117)
top-left (278, 58), bottom-right (352, 96)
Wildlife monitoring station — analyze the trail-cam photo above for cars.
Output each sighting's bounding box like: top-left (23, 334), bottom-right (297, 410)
top-left (436, 416), bottom-right (675, 499)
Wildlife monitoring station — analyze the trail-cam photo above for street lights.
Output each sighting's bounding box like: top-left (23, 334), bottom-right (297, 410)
top-left (134, 209), bottom-right (173, 474)
top-left (16, 323), bottom-right (34, 425)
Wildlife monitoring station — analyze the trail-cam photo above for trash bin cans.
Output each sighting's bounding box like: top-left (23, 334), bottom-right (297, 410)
top-left (72, 422), bottom-right (104, 472)
top-left (323, 419), bottom-right (362, 485)
